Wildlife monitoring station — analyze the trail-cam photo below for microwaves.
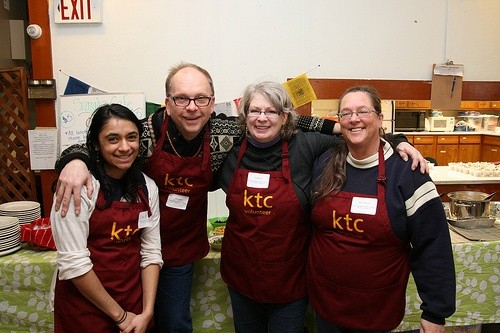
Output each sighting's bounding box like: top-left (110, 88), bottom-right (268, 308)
top-left (425, 116), bottom-right (454, 132)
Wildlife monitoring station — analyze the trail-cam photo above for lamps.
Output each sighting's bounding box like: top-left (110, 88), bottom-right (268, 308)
top-left (27, 79), bottom-right (57, 100)
top-left (26, 24), bottom-right (42, 40)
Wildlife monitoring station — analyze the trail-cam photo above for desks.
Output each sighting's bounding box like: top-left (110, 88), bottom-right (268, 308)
top-left (429, 166), bottom-right (500, 186)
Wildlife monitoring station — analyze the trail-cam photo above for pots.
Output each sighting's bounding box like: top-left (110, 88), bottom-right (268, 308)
top-left (447, 190), bottom-right (492, 220)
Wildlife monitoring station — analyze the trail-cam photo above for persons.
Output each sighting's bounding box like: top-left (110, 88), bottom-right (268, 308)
top-left (51, 64), bottom-right (342, 333)
top-left (48, 104), bottom-right (164, 333)
top-left (209, 80), bottom-right (431, 333)
top-left (308, 86), bottom-right (457, 333)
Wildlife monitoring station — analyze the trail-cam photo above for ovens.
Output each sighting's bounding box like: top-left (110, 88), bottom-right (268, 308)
top-left (395, 108), bottom-right (430, 132)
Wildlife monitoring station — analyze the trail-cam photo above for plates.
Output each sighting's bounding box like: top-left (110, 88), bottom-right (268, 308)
top-left (208, 234), bottom-right (225, 248)
top-left (209, 226), bottom-right (227, 238)
top-left (0, 200), bottom-right (42, 257)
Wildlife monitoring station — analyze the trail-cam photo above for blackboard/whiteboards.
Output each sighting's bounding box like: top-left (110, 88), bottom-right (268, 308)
top-left (56, 92), bottom-right (146, 162)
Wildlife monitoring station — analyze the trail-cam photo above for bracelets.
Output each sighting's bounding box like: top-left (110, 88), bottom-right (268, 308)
top-left (116, 311), bottom-right (127, 323)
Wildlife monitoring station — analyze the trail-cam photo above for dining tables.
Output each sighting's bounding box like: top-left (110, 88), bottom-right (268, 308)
top-left (0, 229), bottom-right (500, 333)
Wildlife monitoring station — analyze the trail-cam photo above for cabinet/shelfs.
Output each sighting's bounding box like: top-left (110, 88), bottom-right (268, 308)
top-left (407, 136), bottom-right (500, 163)
top-left (396, 101), bottom-right (500, 111)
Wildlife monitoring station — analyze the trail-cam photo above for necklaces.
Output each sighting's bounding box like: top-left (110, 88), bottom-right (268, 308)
top-left (166, 130), bottom-right (203, 158)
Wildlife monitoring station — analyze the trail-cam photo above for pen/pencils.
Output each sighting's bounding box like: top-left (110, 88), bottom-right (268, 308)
top-left (451, 78), bottom-right (456, 98)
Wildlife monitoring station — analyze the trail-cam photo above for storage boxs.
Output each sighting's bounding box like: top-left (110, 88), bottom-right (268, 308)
top-left (482, 115), bottom-right (500, 132)
top-left (458, 115), bottom-right (483, 129)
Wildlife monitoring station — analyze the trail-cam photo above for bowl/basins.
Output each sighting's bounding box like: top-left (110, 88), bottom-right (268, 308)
top-left (448, 160), bottom-right (500, 176)
top-left (453, 126), bottom-right (469, 131)
top-left (207, 216), bottom-right (228, 228)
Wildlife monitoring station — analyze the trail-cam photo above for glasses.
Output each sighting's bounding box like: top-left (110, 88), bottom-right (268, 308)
top-left (166, 94), bottom-right (213, 108)
top-left (244, 106), bottom-right (283, 118)
top-left (337, 107), bottom-right (380, 121)
